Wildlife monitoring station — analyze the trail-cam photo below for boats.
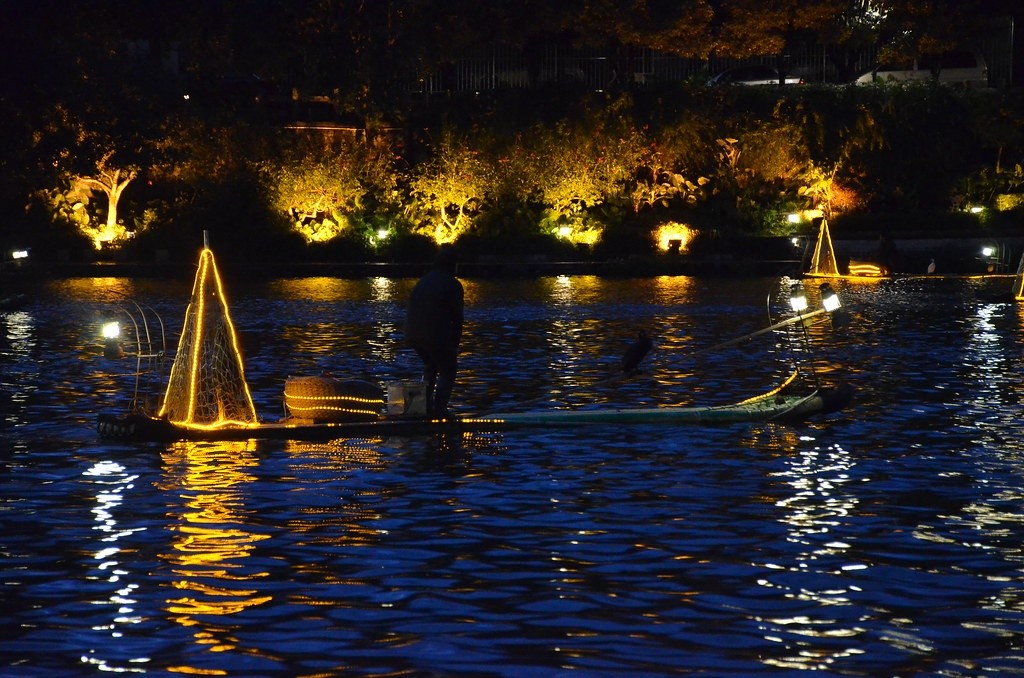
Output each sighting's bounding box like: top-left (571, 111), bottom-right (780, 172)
top-left (800, 203), bottom-right (1024, 282)
top-left (97, 227), bottom-right (852, 448)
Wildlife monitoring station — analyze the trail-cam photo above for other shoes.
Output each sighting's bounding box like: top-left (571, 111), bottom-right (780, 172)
top-left (426, 407), bottom-right (435, 420)
top-left (436, 410), bottom-right (463, 421)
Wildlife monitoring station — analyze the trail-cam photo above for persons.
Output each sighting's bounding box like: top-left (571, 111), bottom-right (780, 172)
top-left (406, 249), bottom-right (464, 422)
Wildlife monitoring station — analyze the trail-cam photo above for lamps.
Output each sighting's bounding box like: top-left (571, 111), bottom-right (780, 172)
top-left (981, 238), bottom-right (1000, 275)
top-left (788, 272), bottom-right (813, 328)
top-left (818, 283), bottom-right (852, 331)
top-left (557, 215), bottom-right (570, 235)
top-left (101, 309), bottom-right (125, 360)
top-left (68, 201), bottom-right (83, 222)
top-left (668, 239), bottom-right (683, 254)
